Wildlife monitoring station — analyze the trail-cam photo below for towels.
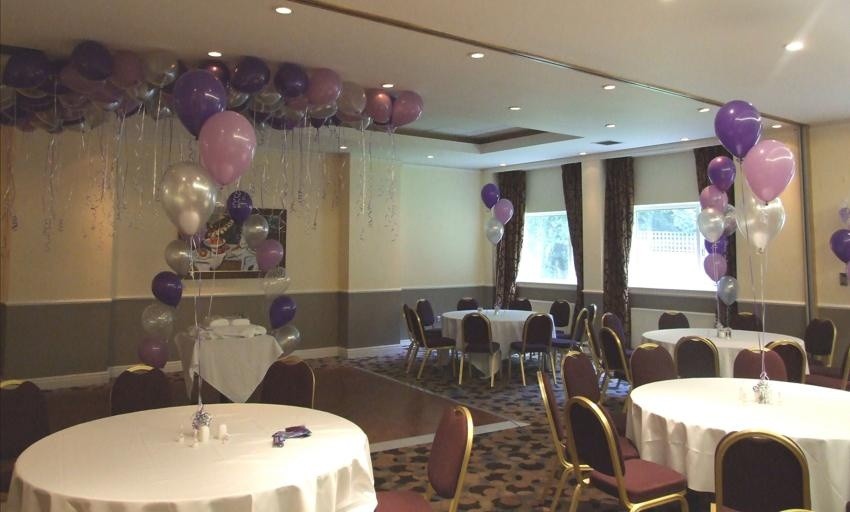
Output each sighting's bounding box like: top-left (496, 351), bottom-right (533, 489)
top-left (214, 324), bottom-right (267, 338)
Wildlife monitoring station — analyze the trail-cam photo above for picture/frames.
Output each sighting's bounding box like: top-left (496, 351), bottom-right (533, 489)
top-left (179, 208), bottom-right (287, 279)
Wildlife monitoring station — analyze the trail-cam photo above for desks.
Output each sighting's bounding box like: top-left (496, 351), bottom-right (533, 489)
top-left (174, 333), bottom-right (283, 405)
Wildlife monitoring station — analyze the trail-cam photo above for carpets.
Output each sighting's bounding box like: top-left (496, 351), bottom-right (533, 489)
top-left (45, 365), bottom-right (529, 453)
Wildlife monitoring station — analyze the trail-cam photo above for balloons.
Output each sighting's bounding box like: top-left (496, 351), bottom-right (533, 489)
top-left (1, 38), bottom-right (423, 139)
top-left (735, 194), bottom-right (788, 256)
top-left (159, 160), bottom-right (221, 237)
top-left (830, 229), bottom-right (850, 264)
top-left (696, 154), bottom-right (738, 308)
top-left (139, 190), bottom-right (303, 370)
top-left (496, 199), bottom-right (515, 223)
top-left (744, 140), bottom-right (795, 203)
top-left (479, 182), bottom-right (501, 211)
top-left (713, 98), bottom-right (763, 161)
top-left (486, 218), bottom-right (505, 246)
top-left (196, 109), bottom-right (259, 189)
